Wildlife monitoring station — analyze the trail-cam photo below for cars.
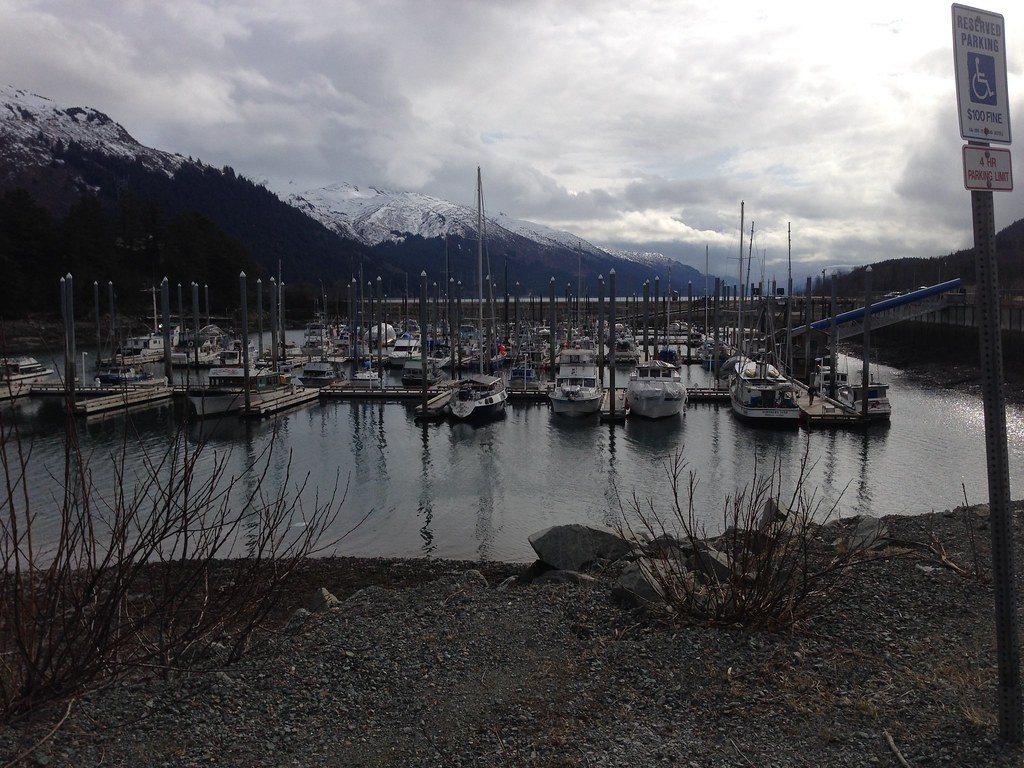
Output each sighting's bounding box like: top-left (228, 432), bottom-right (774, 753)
top-left (883, 291), bottom-right (903, 300)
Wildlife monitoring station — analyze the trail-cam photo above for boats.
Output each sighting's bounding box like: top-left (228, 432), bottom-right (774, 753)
top-left (0, 355), bottom-right (55, 401)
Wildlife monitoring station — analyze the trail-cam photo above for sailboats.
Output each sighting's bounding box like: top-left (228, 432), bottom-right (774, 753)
top-left (90, 164), bottom-right (894, 428)
